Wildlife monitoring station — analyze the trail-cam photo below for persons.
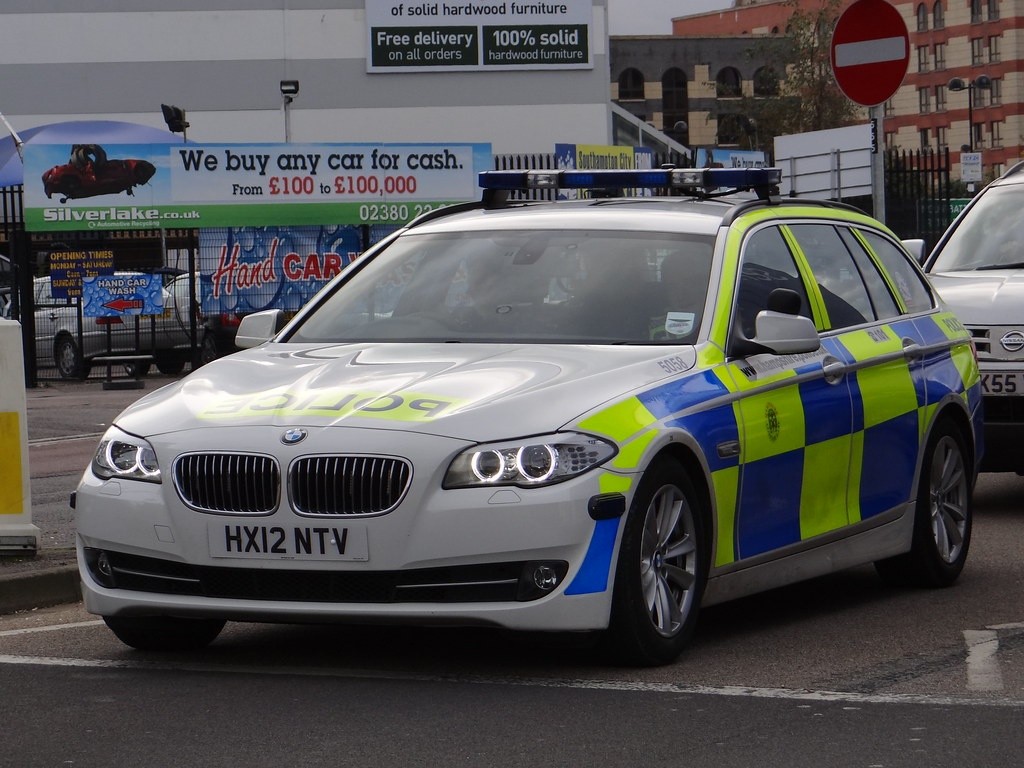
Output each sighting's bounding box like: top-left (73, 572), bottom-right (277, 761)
top-left (655, 250), bottom-right (712, 316)
top-left (460, 244), bottom-right (551, 337)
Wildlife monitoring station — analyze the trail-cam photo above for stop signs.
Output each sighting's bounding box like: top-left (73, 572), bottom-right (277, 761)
top-left (828, 1), bottom-right (908, 109)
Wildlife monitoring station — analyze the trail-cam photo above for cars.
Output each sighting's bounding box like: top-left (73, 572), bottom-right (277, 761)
top-left (66, 165), bottom-right (988, 672)
top-left (2, 267), bottom-right (184, 382)
top-left (923, 158), bottom-right (1024, 483)
top-left (161, 272), bottom-right (201, 375)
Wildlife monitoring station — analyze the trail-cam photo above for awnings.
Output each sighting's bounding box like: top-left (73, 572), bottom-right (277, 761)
top-left (0, 119), bottom-right (199, 187)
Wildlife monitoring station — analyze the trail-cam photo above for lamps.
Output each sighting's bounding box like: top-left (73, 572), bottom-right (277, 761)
top-left (280, 80), bottom-right (299, 103)
